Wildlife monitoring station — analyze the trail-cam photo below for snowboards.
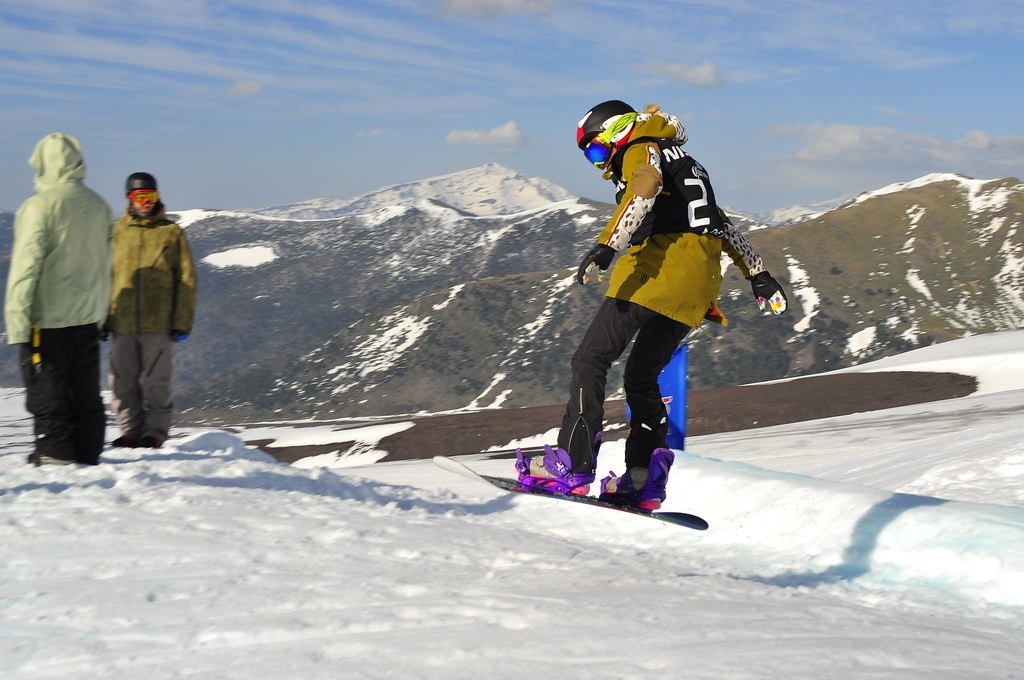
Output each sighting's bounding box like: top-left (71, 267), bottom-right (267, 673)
top-left (432, 455), bottom-right (709, 530)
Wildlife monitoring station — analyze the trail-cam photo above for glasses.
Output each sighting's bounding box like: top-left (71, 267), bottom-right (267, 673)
top-left (584, 112), bottom-right (638, 170)
top-left (128, 190), bottom-right (159, 204)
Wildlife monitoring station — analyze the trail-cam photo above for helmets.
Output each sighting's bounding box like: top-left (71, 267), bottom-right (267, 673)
top-left (577, 100), bottom-right (637, 151)
top-left (126, 172), bottom-right (157, 196)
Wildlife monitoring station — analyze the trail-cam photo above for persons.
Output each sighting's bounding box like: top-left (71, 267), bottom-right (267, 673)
top-left (515, 100), bottom-right (789, 510)
top-left (4, 133), bottom-right (114, 467)
top-left (99, 172), bottom-right (198, 448)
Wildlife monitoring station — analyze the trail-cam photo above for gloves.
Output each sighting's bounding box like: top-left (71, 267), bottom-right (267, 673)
top-left (577, 244), bottom-right (616, 285)
top-left (745, 271), bottom-right (788, 316)
top-left (171, 329), bottom-right (189, 343)
top-left (98, 325), bottom-right (110, 342)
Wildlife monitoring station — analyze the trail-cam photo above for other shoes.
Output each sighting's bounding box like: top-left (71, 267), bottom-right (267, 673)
top-left (29, 452), bottom-right (76, 465)
top-left (112, 436), bottom-right (161, 448)
top-left (530, 456), bottom-right (559, 478)
top-left (605, 467), bottom-right (649, 491)
